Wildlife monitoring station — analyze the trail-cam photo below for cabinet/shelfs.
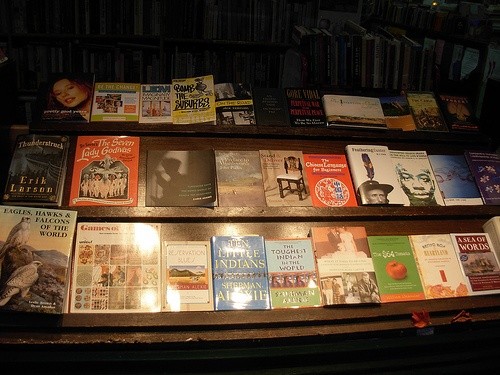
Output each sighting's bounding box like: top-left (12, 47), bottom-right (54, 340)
top-left (0, 0), bottom-right (500, 375)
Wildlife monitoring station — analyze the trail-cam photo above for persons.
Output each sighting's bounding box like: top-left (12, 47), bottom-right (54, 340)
top-left (270, 277), bottom-right (280, 287)
top-left (294, 276), bottom-right (304, 287)
top-left (282, 276), bottom-right (292, 287)
top-left (357, 180), bottom-right (394, 204)
top-left (397, 159), bottom-right (437, 206)
top-left (52, 76), bottom-right (93, 120)
top-left (358, 272), bottom-right (378, 302)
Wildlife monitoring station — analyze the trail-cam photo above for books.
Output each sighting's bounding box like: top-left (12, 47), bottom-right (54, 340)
top-left (0, 0), bottom-right (500, 124)
top-left (0, 74), bottom-right (500, 313)
